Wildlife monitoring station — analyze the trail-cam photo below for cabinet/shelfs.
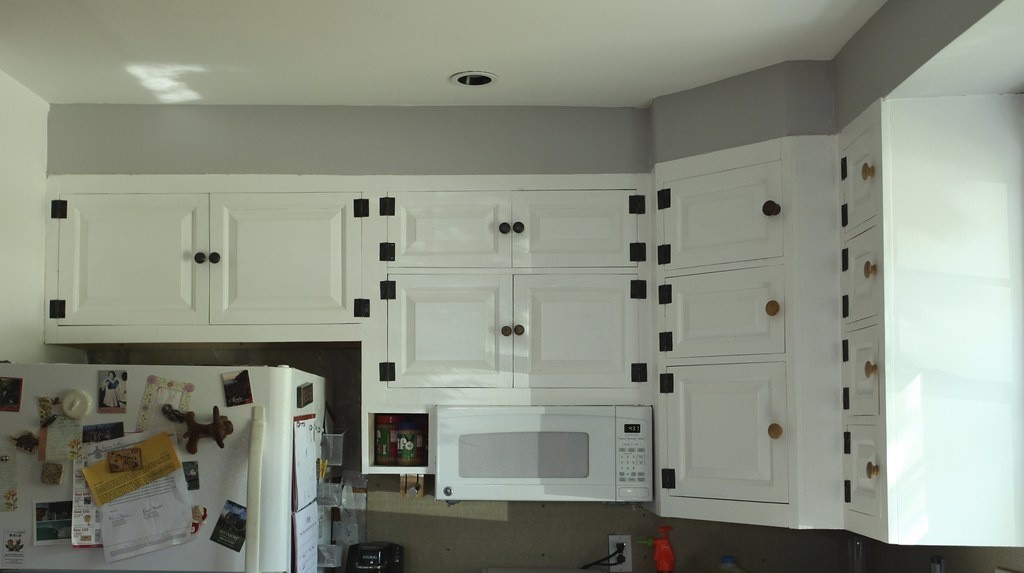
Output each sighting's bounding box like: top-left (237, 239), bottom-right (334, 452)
top-left (46, 172), bottom-right (362, 343)
top-left (361, 173), bottom-right (657, 409)
top-left (362, 408), bottom-right (437, 476)
top-left (651, 132), bottom-right (843, 530)
top-left (840, 98), bottom-right (1024, 547)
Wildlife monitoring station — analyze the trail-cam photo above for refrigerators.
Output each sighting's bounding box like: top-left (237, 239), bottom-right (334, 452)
top-left (0, 363), bottom-right (326, 573)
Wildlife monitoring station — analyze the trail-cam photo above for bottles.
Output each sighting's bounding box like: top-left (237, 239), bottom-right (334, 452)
top-left (373, 415), bottom-right (425, 465)
top-left (718, 557), bottom-right (744, 573)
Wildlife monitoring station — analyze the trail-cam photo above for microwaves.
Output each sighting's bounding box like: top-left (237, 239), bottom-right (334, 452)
top-left (434, 407), bottom-right (654, 502)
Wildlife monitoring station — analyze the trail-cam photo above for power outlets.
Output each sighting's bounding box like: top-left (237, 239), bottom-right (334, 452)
top-left (608, 535), bottom-right (632, 572)
